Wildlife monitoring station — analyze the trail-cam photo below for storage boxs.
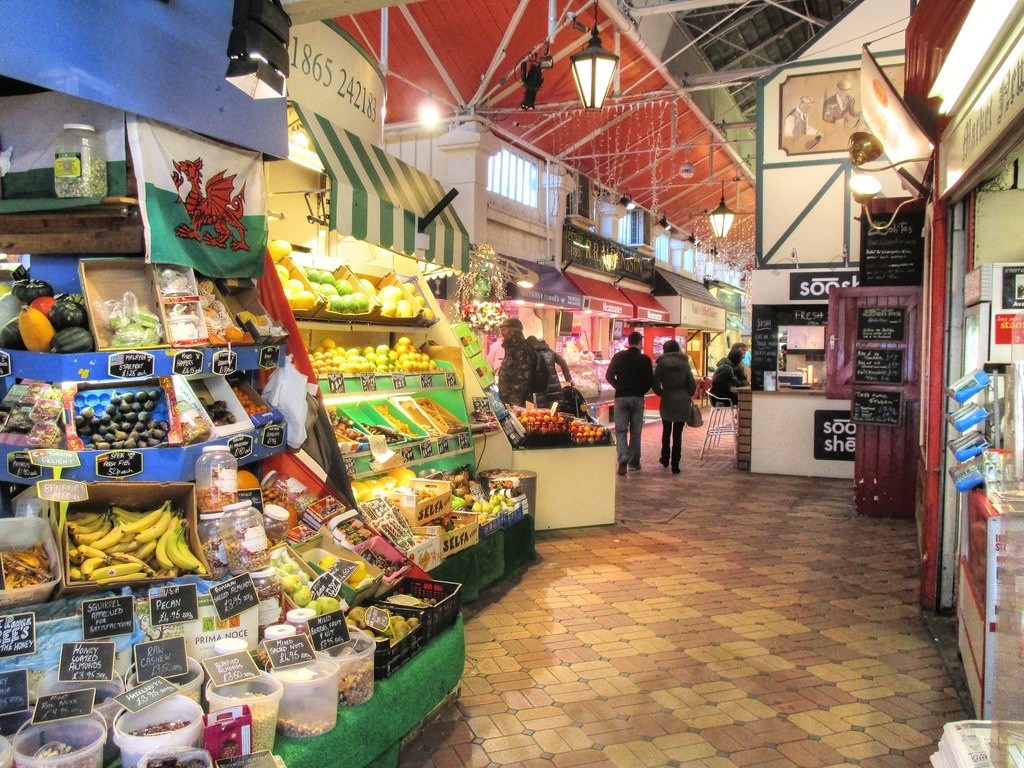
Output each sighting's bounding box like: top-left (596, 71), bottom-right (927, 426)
top-left (328, 508), bottom-right (358, 532)
top-left (0, 479), bottom-right (257, 705)
top-left (324, 395), bottom-right (467, 454)
top-left (273, 256), bottom-right (324, 317)
top-left (778, 372), bottom-right (803, 385)
top-left (302, 517), bottom-right (320, 531)
top-left (354, 536), bottom-right (411, 598)
top-left (0, 370), bottom-right (284, 451)
top-left (163, 299), bottom-right (210, 349)
top-left (341, 528), bottom-right (373, 551)
top-left (469, 352), bottom-right (496, 389)
top-left (450, 322), bottom-right (482, 359)
top-left (333, 518), bottom-right (364, 540)
top-left (0, 262), bottom-right (27, 282)
top-left (267, 542), bottom-right (349, 624)
top-left (303, 512), bottom-right (321, 528)
top-left (76, 256), bottom-right (172, 352)
top-left (568, 432), bottom-right (613, 446)
top-left (196, 274), bottom-right (255, 348)
top-left (346, 264), bottom-right (424, 324)
top-left (154, 261), bottom-right (200, 300)
top-left (479, 492), bottom-right (528, 538)
top-left (368, 576), bottom-right (463, 640)
top-left (344, 597), bottom-right (428, 682)
top-left (411, 511), bottom-right (478, 560)
top-left (392, 269), bottom-right (440, 329)
top-left (399, 536), bottom-right (442, 573)
top-left (290, 534), bottom-right (384, 608)
top-left (387, 477), bottom-right (452, 527)
top-left (301, 263), bottom-right (379, 320)
top-left (214, 275), bottom-right (292, 347)
top-left (306, 494), bottom-right (347, 524)
top-left (484, 385), bottom-right (511, 422)
top-left (501, 417), bottom-right (527, 448)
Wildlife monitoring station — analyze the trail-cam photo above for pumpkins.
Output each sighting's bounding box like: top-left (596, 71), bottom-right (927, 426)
top-left (0, 276), bottom-right (95, 355)
top-left (350, 467), bottom-right (417, 503)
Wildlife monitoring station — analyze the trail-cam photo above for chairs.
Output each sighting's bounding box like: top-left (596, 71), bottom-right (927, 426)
top-left (699, 388), bottom-right (738, 460)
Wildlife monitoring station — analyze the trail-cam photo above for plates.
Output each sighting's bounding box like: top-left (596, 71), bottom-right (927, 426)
top-left (788, 385), bottom-right (812, 388)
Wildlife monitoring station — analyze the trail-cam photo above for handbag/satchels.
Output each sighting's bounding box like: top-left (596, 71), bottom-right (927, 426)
top-left (687, 397), bottom-right (703, 427)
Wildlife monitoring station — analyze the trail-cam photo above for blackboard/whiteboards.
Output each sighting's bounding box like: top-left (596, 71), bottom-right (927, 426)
top-left (262, 633), bottom-right (317, 669)
top-left (856, 306), bottom-right (907, 342)
top-left (0, 668), bottom-right (29, 716)
top-left (0, 612), bottom-right (36, 658)
top-left (57, 642), bottom-right (116, 682)
top-left (750, 303), bottom-right (828, 391)
top-left (82, 595), bottom-right (135, 641)
top-left (853, 346), bottom-right (905, 386)
top-left (307, 609), bottom-right (351, 652)
top-left (208, 571), bottom-right (260, 622)
top-left (113, 676), bottom-right (181, 714)
top-left (148, 584), bottom-right (199, 626)
top-left (31, 688), bottom-right (96, 725)
top-left (215, 749), bottom-right (278, 768)
top-left (326, 558), bottom-right (360, 584)
top-left (850, 388), bottom-right (903, 427)
top-left (201, 650), bottom-right (262, 687)
top-left (134, 635), bottom-right (189, 683)
top-left (860, 212), bottom-right (924, 286)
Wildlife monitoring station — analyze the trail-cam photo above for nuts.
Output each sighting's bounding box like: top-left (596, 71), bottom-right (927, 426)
top-left (200, 532), bottom-right (269, 581)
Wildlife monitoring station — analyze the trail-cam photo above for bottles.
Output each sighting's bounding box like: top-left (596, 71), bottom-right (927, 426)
top-left (261, 470), bottom-right (318, 520)
top-left (257, 625), bottom-right (296, 671)
top-left (262, 505), bottom-right (290, 546)
top-left (214, 638), bottom-right (248, 656)
top-left (251, 567), bottom-right (281, 625)
top-left (54, 124), bottom-right (108, 198)
top-left (195, 445), bottom-right (238, 514)
top-left (198, 512), bottom-right (229, 578)
top-left (283, 608), bottom-right (317, 646)
top-left (222, 500), bottom-right (271, 576)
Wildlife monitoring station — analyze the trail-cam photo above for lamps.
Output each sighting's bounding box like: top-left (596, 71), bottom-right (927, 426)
top-left (619, 193), bottom-right (636, 210)
top-left (842, 243), bottom-right (849, 268)
top-left (926, 0), bottom-right (1024, 118)
top-left (711, 245), bottom-right (720, 257)
top-left (571, 15), bottom-right (590, 33)
top-left (569, 0), bottom-right (621, 114)
top-left (847, 131), bottom-right (933, 172)
top-left (222, 0), bottom-right (291, 101)
top-left (791, 248), bottom-right (799, 270)
top-left (659, 210), bottom-right (672, 230)
top-left (679, 145), bottom-right (695, 179)
top-left (708, 182), bottom-right (736, 239)
top-left (848, 174), bottom-right (924, 230)
top-left (536, 40), bottom-right (554, 70)
top-left (515, 51), bottom-right (545, 112)
top-left (687, 233), bottom-right (698, 246)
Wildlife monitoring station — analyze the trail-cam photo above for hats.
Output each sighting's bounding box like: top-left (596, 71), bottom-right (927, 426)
top-left (499, 318), bottom-right (523, 330)
top-left (662, 340), bottom-right (680, 354)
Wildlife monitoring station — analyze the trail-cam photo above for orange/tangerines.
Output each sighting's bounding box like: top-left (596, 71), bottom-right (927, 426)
top-left (195, 275), bottom-right (243, 343)
top-left (349, 336), bottom-right (437, 373)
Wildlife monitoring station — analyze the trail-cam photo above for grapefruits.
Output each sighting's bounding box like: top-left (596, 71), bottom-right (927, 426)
top-left (360, 279), bottom-right (434, 320)
top-left (267, 239), bottom-right (315, 310)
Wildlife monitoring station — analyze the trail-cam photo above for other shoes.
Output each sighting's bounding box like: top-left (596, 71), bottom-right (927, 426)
top-left (618, 454), bottom-right (628, 475)
top-left (629, 461), bottom-right (640, 470)
top-left (672, 465), bottom-right (680, 474)
top-left (659, 457), bottom-right (669, 467)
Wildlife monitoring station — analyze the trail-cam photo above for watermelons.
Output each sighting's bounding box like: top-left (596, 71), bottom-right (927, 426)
top-left (299, 265), bottom-right (371, 314)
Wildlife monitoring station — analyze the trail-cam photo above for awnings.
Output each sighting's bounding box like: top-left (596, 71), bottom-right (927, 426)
top-left (654, 266), bottom-right (726, 334)
top-left (726, 317), bottom-right (749, 331)
top-left (564, 271), bottom-right (633, 319)
top-left (618, 285), bottom-right (669, 323)
top-left (498, 253), bottom-right (584, 311)
top-left (271, 99), bottom-right (470, 275)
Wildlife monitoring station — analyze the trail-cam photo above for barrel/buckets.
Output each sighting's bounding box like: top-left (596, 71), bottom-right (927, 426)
top-left (265, 651), bottom-right (339, 738)
top-left (37, 663), bottom-right (125, 762)
top-left (113, 694), bottom-right (203, 768)
top-left (125, 656), bottom-right (204, 705)
top-left (478, 469), bottom-right (536, 518)
top-left (12, 709), bottom-right (107, 768)
top-left (0, 735), bottom-right (12, 768)
top-left (206, 669), bottom-right (284, 754)
top-left (308, 625), bottom-right (376, 706)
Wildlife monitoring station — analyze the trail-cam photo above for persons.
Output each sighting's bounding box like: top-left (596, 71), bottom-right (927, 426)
top-left (527, 336), bottom-right (574, 407)
top-left (498, 318), bottom-right (534, 406)
top-left (606, 332), bottom-right (654, 474)
top-left (710, 350), bottom-right (749, 407)
top-left (564, 333), bottom-right (592, 362)
top-left (487, 334), bottom-right (505, 376)
top-left (732, 343), bottom-right (751, 382)
top-left (652, 339), bottom-right (696, 473)
top-left (781, 344), bottom-right (787, 371)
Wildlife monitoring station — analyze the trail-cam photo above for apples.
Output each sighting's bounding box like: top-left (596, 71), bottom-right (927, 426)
top-left (516, 411), bottom-right (606, 444)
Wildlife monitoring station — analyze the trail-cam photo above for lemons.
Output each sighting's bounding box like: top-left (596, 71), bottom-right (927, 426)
top-left (317, 557), bottom-right (374, 588)
top-left (306, 339), bottom-right (375, 376)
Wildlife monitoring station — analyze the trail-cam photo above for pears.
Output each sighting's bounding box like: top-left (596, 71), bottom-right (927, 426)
top-left (269, 556), bottom-right (341, 615)
top-left (345, 607), bottom-right (420, 647)
top-left (471, 493), bottom-right (516, 516)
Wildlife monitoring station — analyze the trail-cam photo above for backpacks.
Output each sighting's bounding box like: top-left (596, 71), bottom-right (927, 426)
top-left (512, 340), bottom-right (549, 393)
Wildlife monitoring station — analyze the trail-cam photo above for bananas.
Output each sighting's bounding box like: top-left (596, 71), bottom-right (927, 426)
top-left (62, 498), bottom-right (207, 584)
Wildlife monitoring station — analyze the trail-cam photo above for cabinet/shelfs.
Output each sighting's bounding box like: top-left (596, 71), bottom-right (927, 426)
top-left (957, 488), bottom-right (1024, 722)
top-left (0, 251), bottom-right (467, 768)
top-left (293, 316), bottom-right (474, 482)
top-left (942, 368), bottom-right (1000, 494)
